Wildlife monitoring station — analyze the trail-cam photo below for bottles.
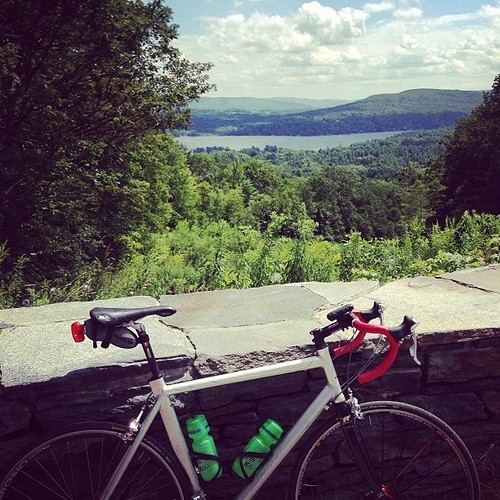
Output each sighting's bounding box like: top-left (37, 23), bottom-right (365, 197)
top-left (231, 417), bottom-right (284, 481)
top-left (185, 411), bottom-right (222, 482)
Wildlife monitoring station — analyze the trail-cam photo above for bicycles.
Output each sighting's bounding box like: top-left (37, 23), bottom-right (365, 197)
top-left (0, 300), bottom-right (480, 500)
